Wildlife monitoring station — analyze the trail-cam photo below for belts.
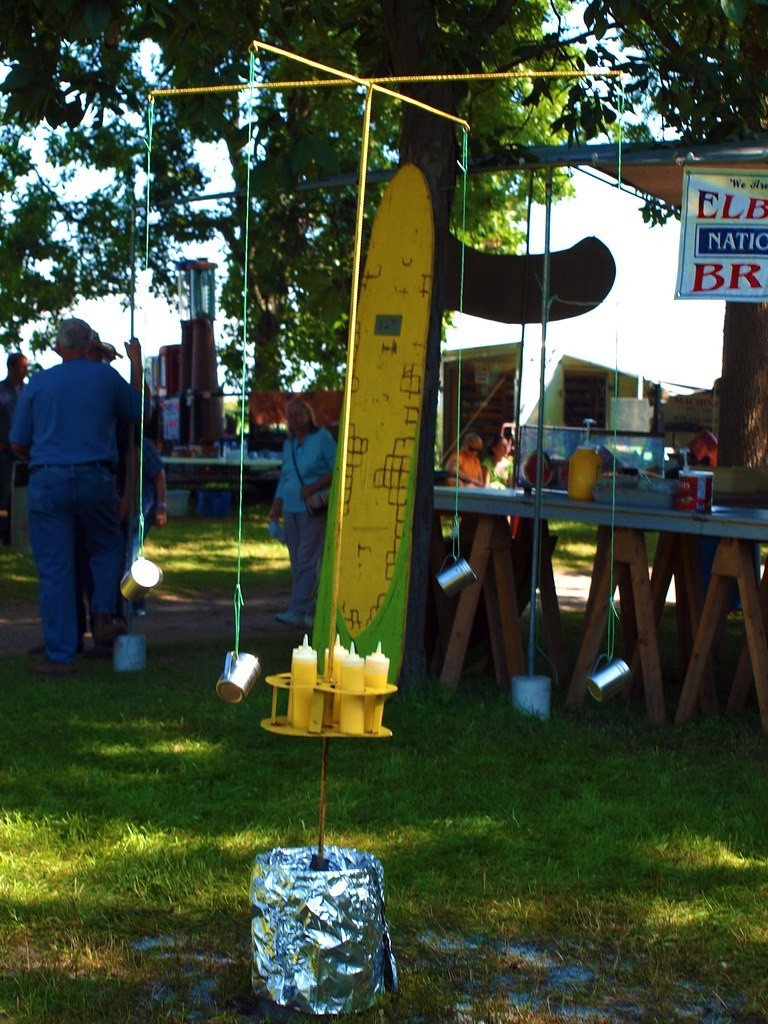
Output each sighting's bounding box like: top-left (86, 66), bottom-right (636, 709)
top-left (28, 462), bottom-right (117, 475)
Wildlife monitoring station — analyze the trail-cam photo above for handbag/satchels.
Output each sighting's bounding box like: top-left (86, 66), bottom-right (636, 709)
top-left (306, 489), bottom-right (330, 518)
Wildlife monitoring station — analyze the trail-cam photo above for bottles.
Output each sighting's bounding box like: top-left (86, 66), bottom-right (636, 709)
top-left (286, 633), bottom-right (318, 729)
top-left (324, 633), bottom-right (391, 735)
top-left (213, 434), bottom-right (249, 460)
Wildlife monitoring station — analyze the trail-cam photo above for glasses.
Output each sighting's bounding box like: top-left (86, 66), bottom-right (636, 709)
top-left (468, 445), bottom-right (482, 454)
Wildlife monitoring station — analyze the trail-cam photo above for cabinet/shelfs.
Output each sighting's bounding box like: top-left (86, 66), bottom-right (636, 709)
top-left (462, 373), bottom-right (606, 438)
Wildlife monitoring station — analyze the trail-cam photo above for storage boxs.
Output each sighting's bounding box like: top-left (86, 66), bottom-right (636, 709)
top-left (198, 490), bottom-right (231, 517)
top-left (167, 490), bottom-right (191, 517)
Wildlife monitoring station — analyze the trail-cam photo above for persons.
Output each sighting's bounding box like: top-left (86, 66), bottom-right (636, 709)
top-left (0, 353), bottom-right (27, 550)
top-left (9, 319), bottom-right (151, 676)
top-left (269, 397), bottom-right (337, 631)
top-left (481, 436), bottom-right (513, 490)
top-left (28, 328), bottom-right (168, 656)
top-left (444, 435), bottom-right (484, 487)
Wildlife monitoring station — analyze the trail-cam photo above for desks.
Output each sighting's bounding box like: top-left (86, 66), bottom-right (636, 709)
top-left (432, 486), bottom-right (768, 730)
top-left (162, 456), bottom-right (283, 485)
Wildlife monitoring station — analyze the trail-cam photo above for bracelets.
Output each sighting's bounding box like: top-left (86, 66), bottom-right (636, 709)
top-left (159, 503), bottom-right (167, 508)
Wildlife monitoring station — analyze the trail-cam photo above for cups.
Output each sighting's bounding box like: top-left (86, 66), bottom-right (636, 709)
top-left (215, 651), bottom-right (262, 704)
top-left (120, 556), bottom-right (163, 602)
top-left (434, 554), bottom-right (479, 598)
top-left (584, 654), bottom-right (633, 703)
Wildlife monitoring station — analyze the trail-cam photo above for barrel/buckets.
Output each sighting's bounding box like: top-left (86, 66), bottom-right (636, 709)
top-left (678, 471), bottom-right (714, 511)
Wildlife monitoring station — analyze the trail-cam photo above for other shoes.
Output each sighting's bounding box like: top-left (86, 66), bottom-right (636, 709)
top-left (275, 610), bottom-right (306, 627)
top-left (93, 613), bottom-right (124, 636)
top-left (35, 659), bottom-right (75, 674)
top-left (135, 608), bottom-right (147, 617)
top-left (304, 614), bottom-right (314, 627)
top-left (27, 637), bottom-right (84, 654)
